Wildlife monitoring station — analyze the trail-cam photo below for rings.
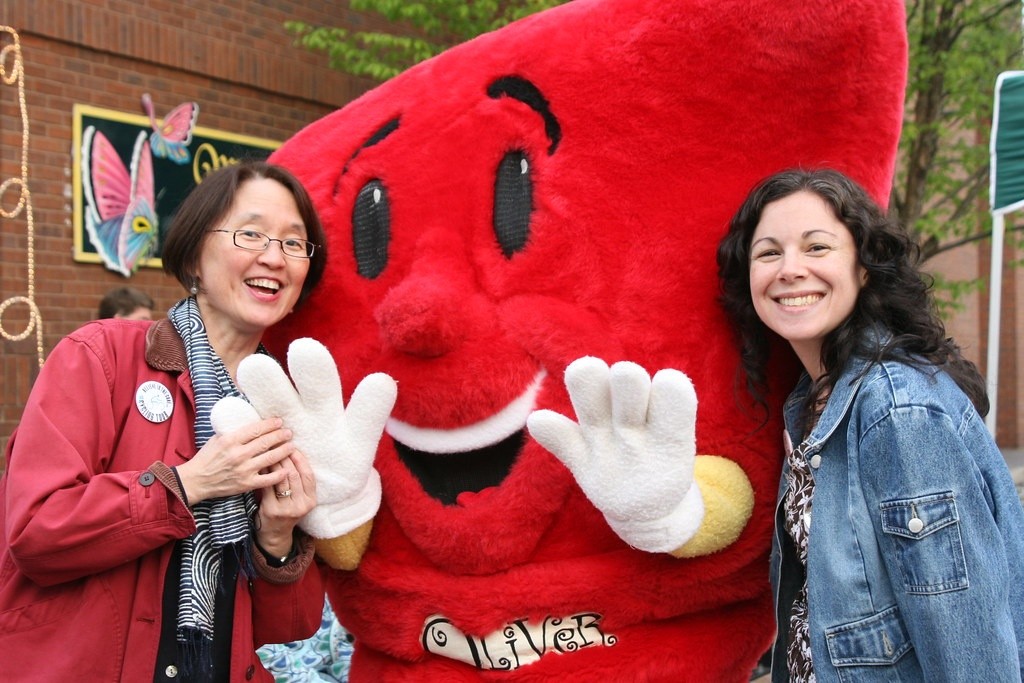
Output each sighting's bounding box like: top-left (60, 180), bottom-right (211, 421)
top-left (277, 490), bottom-right (291, 498)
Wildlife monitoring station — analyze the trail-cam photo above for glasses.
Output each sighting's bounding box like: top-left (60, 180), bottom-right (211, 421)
top-left (208, 227), bottom-right (318, 259)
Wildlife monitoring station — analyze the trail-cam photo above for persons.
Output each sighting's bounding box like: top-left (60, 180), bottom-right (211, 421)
top-left (716, 169), bottom-right (1024, 683)
top-left (0, 160), bottom-right (326, 683)
top-left (98, 285), bottom-right (153, 322)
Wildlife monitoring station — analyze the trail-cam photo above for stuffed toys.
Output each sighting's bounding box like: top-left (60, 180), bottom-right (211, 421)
top-left (265, 0), bottom-right (909, 683)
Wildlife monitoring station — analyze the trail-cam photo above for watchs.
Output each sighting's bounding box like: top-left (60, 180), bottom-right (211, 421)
top-left (253, 542), bottom-right (297, 568)
